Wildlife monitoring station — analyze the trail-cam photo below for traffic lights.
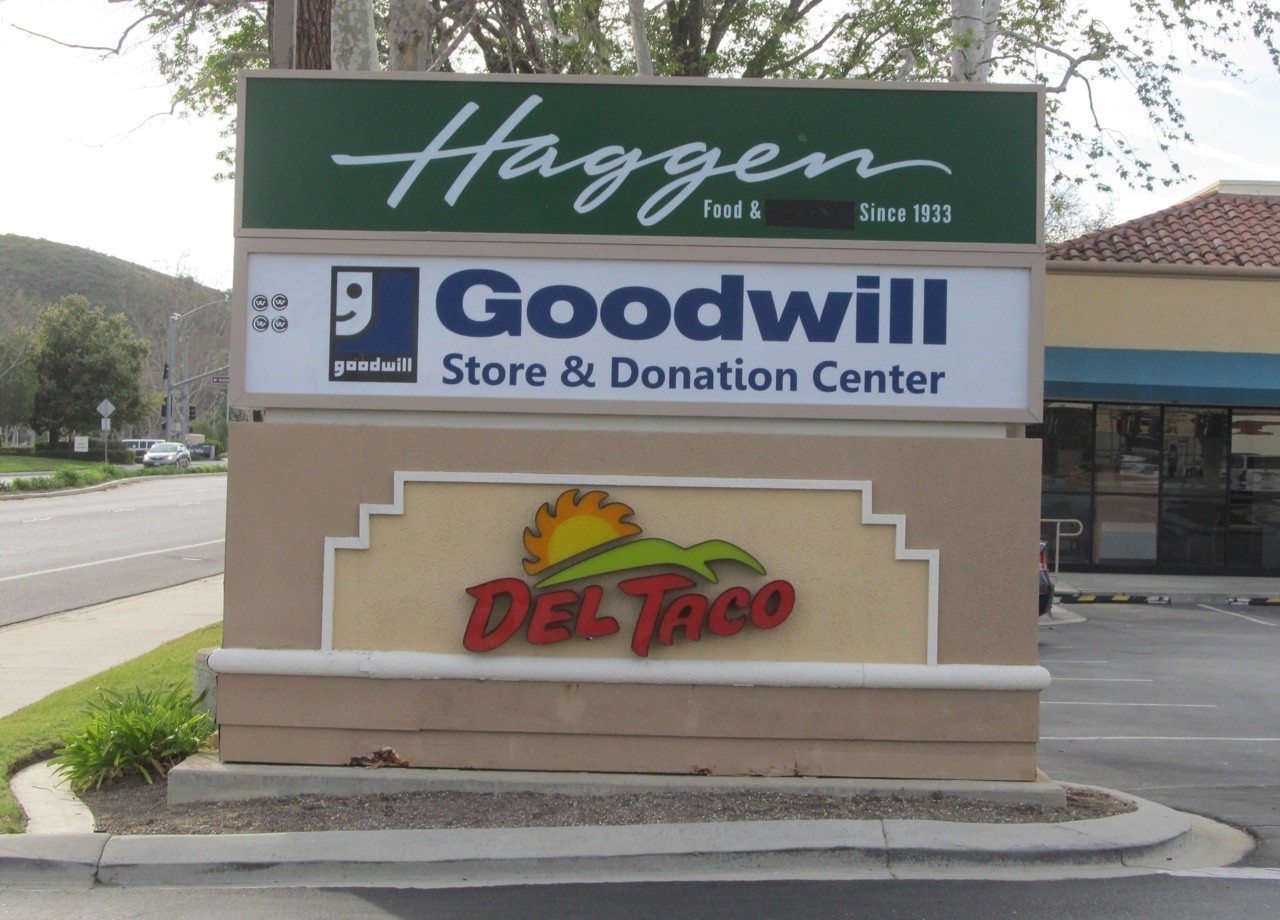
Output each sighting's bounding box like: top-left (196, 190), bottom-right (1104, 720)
top-left (162, 360), bottom-right (169, 379)
top-left (187, 405), bottom-right (197, 422)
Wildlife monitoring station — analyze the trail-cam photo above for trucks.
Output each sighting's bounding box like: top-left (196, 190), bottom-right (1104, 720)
top-left (1111, 432), bottom-right (1203, 481)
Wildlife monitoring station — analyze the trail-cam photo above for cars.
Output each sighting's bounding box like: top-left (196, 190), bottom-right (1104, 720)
top-left (1039, 540), bottom-right (1054, 617)
top-left (143, 442), bottom-right (192, 469)
top-left (1230, 454), bottom-right (1280, 494)
